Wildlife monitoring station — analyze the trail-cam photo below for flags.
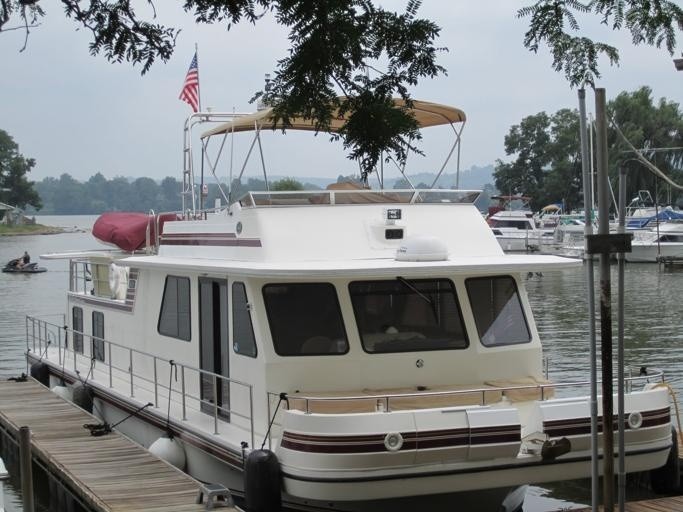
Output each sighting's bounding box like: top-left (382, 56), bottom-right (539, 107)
top-left (179, 52), bottom-right (199, 112)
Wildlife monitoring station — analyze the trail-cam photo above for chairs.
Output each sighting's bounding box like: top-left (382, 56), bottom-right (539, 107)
top-left (91, 210), bottom-right (178, 253)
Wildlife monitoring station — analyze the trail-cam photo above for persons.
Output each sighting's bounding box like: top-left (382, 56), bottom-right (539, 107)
top-left (18, 252), bottom-right (30, 269)
